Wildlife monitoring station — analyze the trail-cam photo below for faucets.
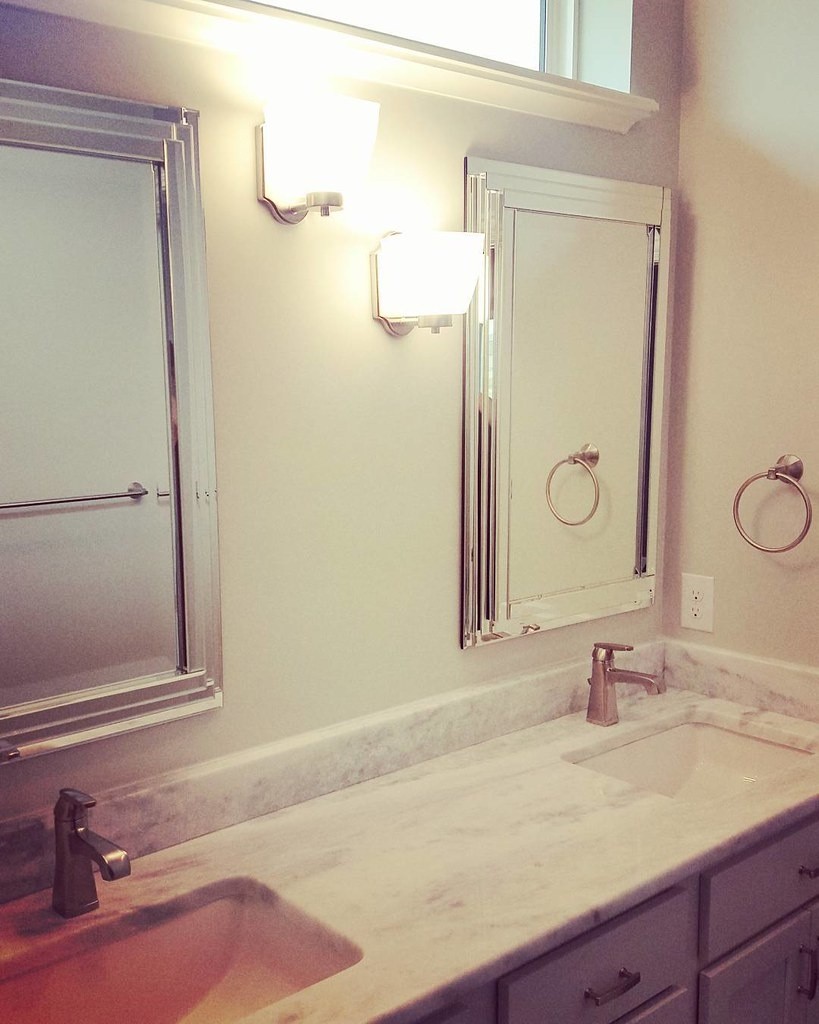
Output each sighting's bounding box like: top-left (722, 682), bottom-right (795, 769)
top-left (52, 787), bottom-right (132, 919)
top-left (586, 642), bottom-right (668, 727)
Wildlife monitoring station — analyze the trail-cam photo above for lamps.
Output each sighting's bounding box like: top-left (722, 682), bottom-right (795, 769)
top-left (369, 230), bottom-right (486, 337)
top-left (255, 100), bottom-right (381, 225)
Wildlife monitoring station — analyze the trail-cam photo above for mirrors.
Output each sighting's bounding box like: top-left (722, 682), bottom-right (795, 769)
top-left (460, 155), bottom-right (671, 652)
top-left (0, 78), bottom-right (224, 772)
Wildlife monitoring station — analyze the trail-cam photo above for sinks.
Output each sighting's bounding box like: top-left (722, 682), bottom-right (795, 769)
top-left (1, 874), bottom-right (366, 1023)
top-left (561, 708), bottom-right (819, 800)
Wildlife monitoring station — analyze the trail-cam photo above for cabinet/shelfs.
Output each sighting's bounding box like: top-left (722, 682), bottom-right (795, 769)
top-left (429, 812), bottom-right (819, 1024)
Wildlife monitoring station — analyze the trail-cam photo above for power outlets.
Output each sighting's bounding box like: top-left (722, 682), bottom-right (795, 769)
top-left (682, 570), bottom-right (715, 630)
